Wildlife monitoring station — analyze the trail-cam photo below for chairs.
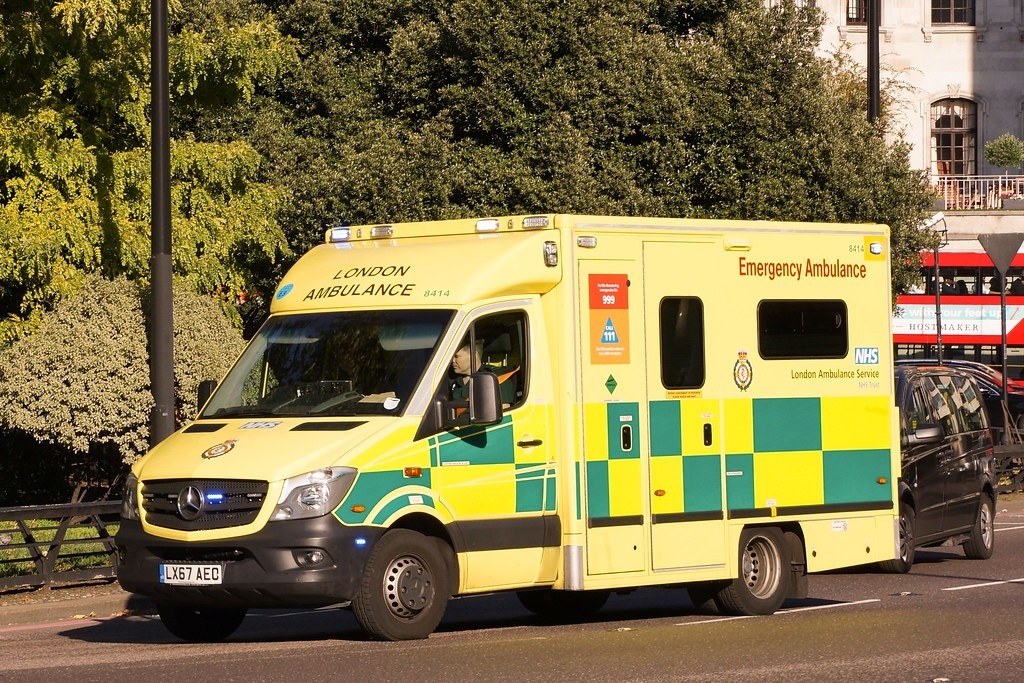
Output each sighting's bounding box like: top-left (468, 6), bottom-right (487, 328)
top-left (929, 278), bottom-right (1024, 295)
top-left (481, 331), bottom-right (522, 407)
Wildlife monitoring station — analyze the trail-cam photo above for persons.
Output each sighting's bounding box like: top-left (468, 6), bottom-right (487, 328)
top-left (441, 339), bottom-right (513, 426)
top-left (943, 280), bottom-right (968, 294)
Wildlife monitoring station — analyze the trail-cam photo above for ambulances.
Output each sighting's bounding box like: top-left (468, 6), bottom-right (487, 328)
top-left (114, 212), bottom-right (899, 644)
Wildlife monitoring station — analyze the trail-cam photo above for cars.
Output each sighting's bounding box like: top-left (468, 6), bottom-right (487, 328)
top-left (877, 364), bottom-right (998, 574)
top-left (894, 360), bottom-right (1024, 446)
